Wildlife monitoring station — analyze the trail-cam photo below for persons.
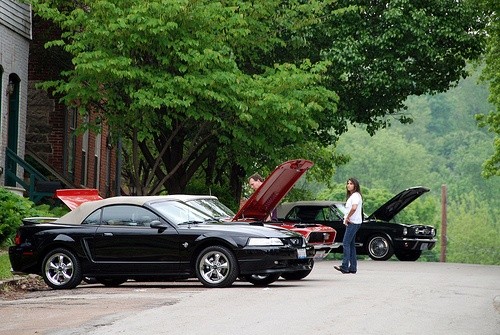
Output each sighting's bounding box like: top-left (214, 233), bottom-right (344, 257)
top-left (243, 173), bottom-right (278, 222)
top-left (333, 178), bottom-right (362, 273)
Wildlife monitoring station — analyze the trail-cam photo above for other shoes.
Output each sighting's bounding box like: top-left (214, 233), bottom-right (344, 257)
top-left (334, 266), bottom-right (349, 274)
top-left (349, 271), bottom-right (356, 274)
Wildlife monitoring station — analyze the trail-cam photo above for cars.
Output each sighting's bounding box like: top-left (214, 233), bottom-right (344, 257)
top-left (272, 185), bottom-right (437, 262)
top-left (54, 159), bottom-right (340, 279)
top-left (8, 196), bottom-right (316, 289)
